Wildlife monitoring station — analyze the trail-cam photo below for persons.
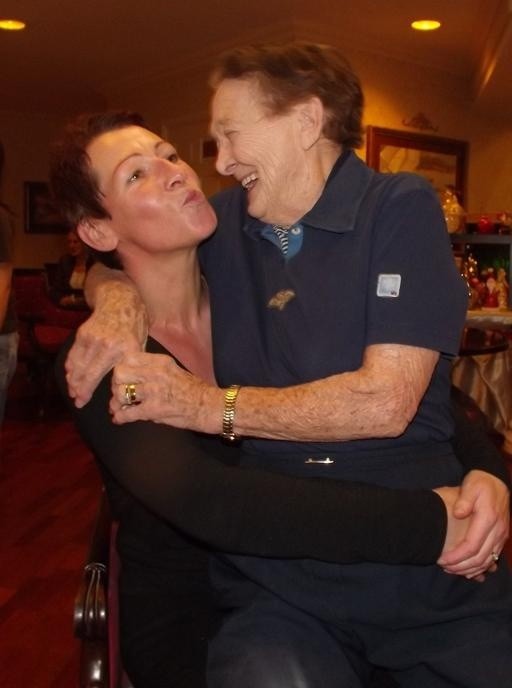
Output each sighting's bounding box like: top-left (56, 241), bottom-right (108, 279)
top-left (42, 227), bottom-right (109, 315)
top-left (66, 33), bottom-right (512, 687)
top-left (38, 109), bottom-right (511, 688)
top-left (0, 201), bottom-right (21, 431)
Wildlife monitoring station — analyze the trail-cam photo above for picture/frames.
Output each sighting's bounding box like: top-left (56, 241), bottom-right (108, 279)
top-left (366, 123), bottom-right (470, 206)
top-left (21, 180), bottom-right (68, 236)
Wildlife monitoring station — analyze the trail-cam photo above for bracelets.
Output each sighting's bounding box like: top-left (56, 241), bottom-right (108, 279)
top-left (71, 294), bottom-right (76, 306)
top-left (223, 378), bottom-right (241, 445)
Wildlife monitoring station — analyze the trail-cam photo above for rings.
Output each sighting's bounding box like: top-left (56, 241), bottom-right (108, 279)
top-left (124, 381), bottom-right (139, 409)
top-left (490, 551), bottom-right (500, 564)
